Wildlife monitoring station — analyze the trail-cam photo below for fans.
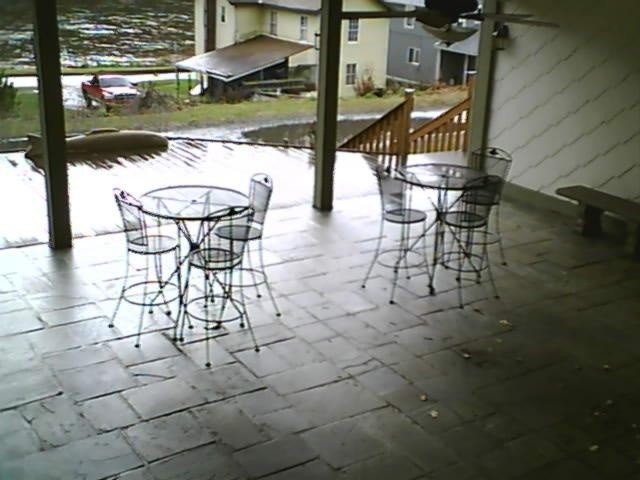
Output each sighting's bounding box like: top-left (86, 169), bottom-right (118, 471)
top-left (341, 1), bottom-right (560, 31)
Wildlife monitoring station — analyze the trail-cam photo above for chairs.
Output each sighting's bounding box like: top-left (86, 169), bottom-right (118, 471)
top-left (362, 146), bottom-right (514, 308)
top-left (108, 171), bottom-right (282, 368)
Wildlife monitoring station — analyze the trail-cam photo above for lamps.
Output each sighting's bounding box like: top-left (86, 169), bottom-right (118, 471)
top-left (421, 20), bottom-right (477, 48)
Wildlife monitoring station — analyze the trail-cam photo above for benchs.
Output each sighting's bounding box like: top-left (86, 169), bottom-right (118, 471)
top-left (553, 186), bottom-right (638, 256)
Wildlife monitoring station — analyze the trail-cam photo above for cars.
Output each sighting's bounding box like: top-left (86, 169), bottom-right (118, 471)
top-left (80, 72), bottom-right (143, 113)
